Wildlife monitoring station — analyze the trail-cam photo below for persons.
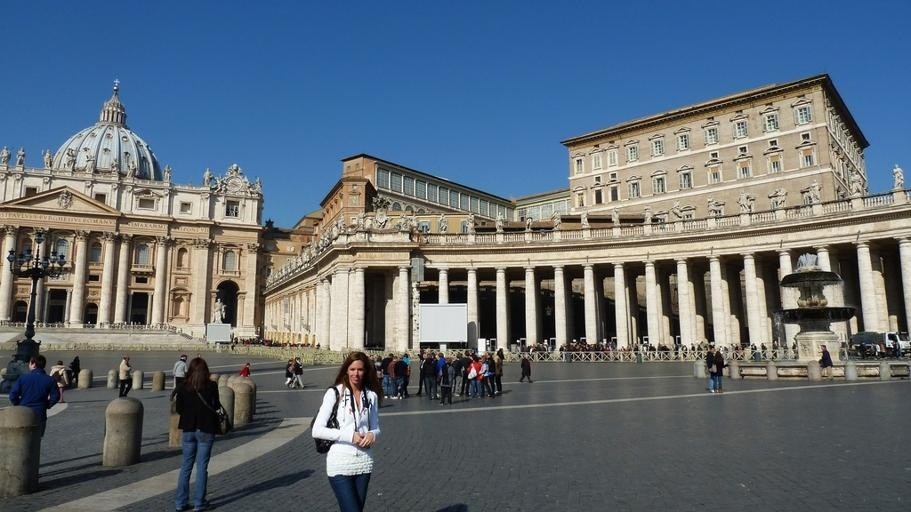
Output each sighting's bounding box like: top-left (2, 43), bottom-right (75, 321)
top-left (818, 345), bottom-right (834, 382)
top-left (705, 345), bottom-right (714, 393)
top-left (310, 351), bottom-right (382, 512)
top-left (172, 357), bottom-right (221, 510)
top-left (519, 355), bottom-right (533, 383)
top-left (283, 356), bottom-right (306, 390)
top-left (371, 346), bottom-right (504, 407)
top-left (712, 352), bottom-right (724, 393)
top-left (169, 354), bottom-right (188, 400)
top-left (49, 361), bottom-right (68, 404)
top-left (856, 339), bottom-right (900, 360)
top-left (70, 354), bottom-right (80, 386)
top-left (196, 352), bottom-right (202, 358)
top-left (7, 355), bottom-right (61, 437)
top-left (118, 354), bottom-right (133, 397)
top-left (519, 341), bottom-right (549, 360)
top-left (557, 339), bottom-right (798, 360)
top-left (240, 363), bottom-right (250, 378)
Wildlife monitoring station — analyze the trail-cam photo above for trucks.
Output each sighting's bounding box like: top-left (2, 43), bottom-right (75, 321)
top-left (852, 330), bottom-right (911, 356)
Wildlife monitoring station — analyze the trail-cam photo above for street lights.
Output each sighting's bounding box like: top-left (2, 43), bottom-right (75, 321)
top-left (1, 230), bottom-right (76, 391)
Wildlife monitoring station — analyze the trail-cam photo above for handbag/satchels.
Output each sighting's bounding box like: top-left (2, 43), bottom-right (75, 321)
top-left (52, 371), bottom-right (62, 382)
top-left (468, 367), bottom-right (478, 380)
top-left (310, 410), bottom-right (339, 453)
top-left (215, 408), bottom-right (231, 435)
